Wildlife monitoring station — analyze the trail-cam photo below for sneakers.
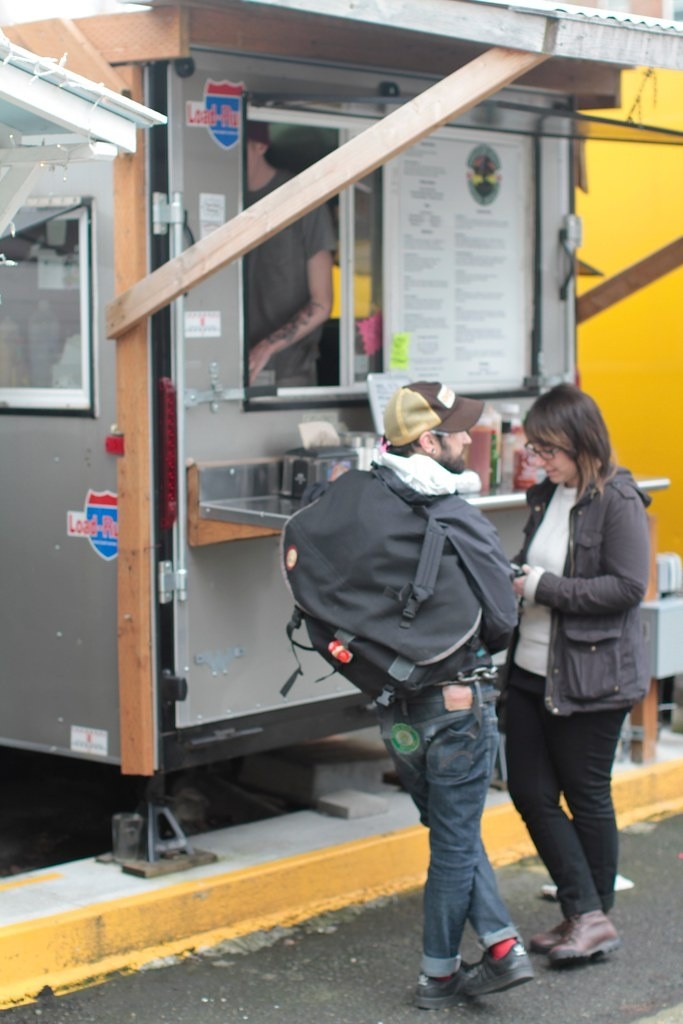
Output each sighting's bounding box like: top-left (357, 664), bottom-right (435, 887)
top-left (415, 959), bottom-right (472, 1008)
top-left (465, 942), bottom-right (533, 997)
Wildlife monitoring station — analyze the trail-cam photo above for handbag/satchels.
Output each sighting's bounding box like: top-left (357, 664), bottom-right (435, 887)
top-left (278, 469), bottom-right (484, 707)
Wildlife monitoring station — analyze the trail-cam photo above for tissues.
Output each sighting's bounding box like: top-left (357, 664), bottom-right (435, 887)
top-left (278, 420), bottom-right (357, 498)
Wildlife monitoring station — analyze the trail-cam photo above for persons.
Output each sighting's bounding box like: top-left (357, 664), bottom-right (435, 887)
top-left (496, 382), bottom-right (651, 964)
top-left (245, 121), bottom-right (337, 385)
top-left (377, 382), bottom-right (537, 1009)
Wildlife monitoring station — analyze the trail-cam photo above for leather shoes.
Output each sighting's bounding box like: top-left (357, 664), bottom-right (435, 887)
top-left (550, 909), bottom-right (621, 968)
top-left (531, 919), bottom-right (575, 952)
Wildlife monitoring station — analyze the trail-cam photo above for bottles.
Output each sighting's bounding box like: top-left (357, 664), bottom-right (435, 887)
top-left (482, 400), bottom-right (524, 486)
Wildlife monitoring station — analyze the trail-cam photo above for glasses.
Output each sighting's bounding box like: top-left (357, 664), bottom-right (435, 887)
top-left (524, 439), bottom-right (569, 461)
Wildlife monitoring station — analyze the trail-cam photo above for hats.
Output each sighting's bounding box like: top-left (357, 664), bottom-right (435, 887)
top-left (382, 380), bottom-right (485, 448)
top-left (244, 121), bottom-right (269, 143)
